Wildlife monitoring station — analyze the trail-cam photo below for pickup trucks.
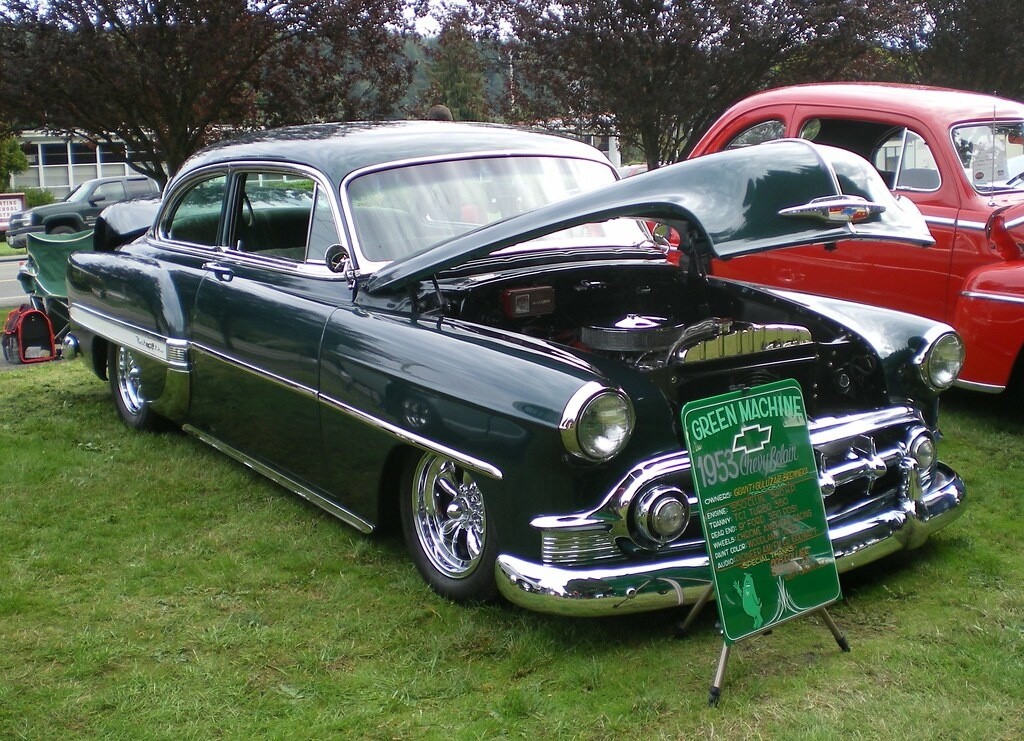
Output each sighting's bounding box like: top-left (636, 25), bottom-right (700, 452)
top-left (5, 175), bottom-right (161, 249)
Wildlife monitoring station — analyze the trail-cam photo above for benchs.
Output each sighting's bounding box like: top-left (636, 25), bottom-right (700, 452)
top-left (223, 223), bottom-right (454, 260)
top-left (169, 204), bottom-right (419, 243)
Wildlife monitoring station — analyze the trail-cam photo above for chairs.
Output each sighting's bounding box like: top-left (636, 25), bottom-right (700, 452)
top-left (16, 228), bottom-right (96, 341)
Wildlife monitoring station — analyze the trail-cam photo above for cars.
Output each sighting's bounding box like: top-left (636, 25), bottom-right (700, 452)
top-left (644, 81), bottom-right (1023, 395)
top-left (62, 119), bottom-right (968, 620)
